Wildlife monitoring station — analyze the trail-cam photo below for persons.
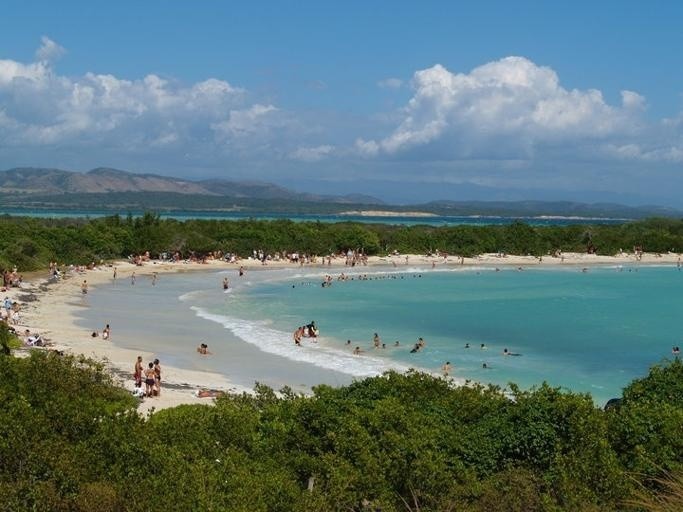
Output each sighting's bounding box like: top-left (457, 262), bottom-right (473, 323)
top-left (321, 272), bottom-right (422, 288)
top-left (49, 258), bottom-right (113, 280)
top-left (2, 264), bottom-right (42, 346)
top-left (495, 248), bottom-right (681, 272)
top-left (671, 346), bottom-right (680, 353)
top-left (131, 271), bottom-right (137, 285)
top-left (250, 247), bottom-right (370, 267)
top-left (293, 320), bottom-right (424, 355)
top-left (387, 248), bottom-right (485, 268)
top-left (113, 267), bottom-right (118, 279)
top-left (133, 356), bottom-right (161, 398)
top-left (81, 280), bottom-right (89, 296)
top-left (127, 248), bottom-right (239, 266)
top-left (223, 278), bottom-right (229, 290)
top-left (194, 389), bottom-right (226, 398)
top-left (197, 343), bottom-right (207, 354)
top-left (441, 343), bottom-right (523, 378)
top-left (239, 265), bottom-right (243, 277)
top-left (92, 324), bottom-right (111, 339)
top-left (150, 275), bottom-right (159, 286)
